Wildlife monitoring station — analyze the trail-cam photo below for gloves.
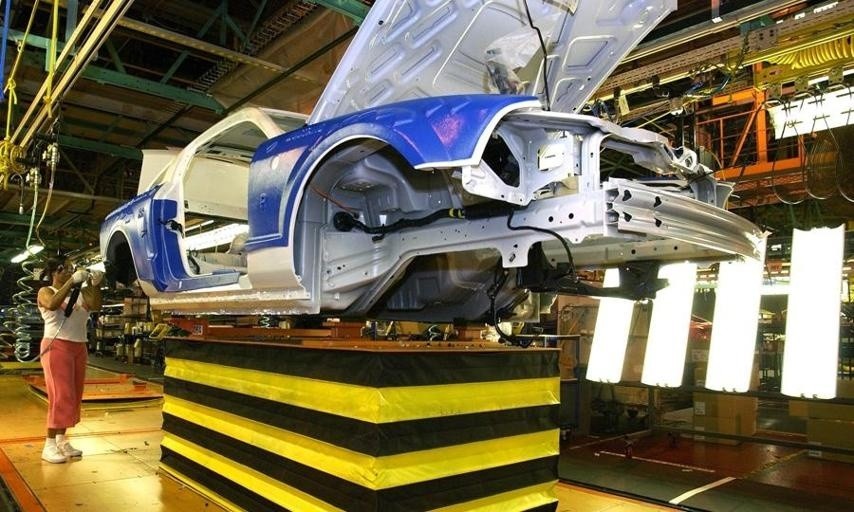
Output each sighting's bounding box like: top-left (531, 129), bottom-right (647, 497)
top-left (72, 270), bottom-right (103, 286)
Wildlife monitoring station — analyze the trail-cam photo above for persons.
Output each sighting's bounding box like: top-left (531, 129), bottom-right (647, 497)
top-left (35, 254), bottom-right (109, 464)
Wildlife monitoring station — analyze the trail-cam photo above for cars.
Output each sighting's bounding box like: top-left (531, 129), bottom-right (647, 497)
top-left (99, 0), bottom-right (765, 323)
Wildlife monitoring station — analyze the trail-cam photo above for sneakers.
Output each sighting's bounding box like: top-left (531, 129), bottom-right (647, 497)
top-left (41, 442), bottom-right (83, 463)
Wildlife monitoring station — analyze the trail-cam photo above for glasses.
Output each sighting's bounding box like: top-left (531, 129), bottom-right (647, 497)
top-left (57, 265), bottom-right (73, 272)
